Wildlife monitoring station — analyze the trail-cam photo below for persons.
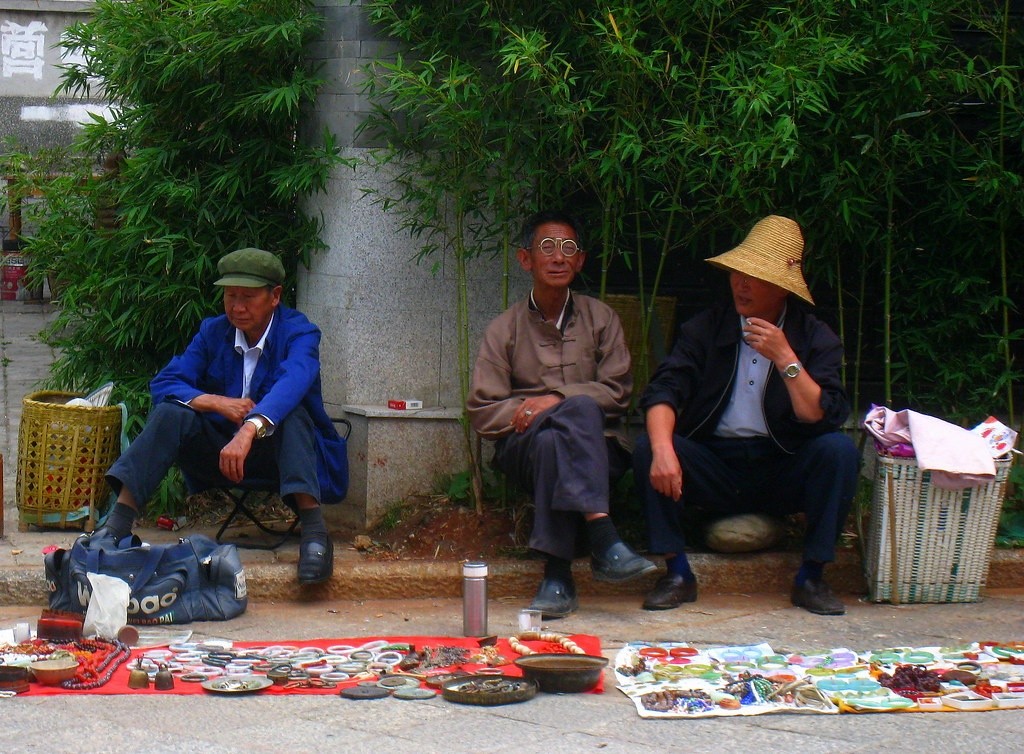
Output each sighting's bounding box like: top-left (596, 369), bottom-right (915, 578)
top-left (465, 210), bottom-right (657, 616)
top-left (98, 248), bottom-right (348, 582)
top-left (632, 215), bottom-right (859, 615)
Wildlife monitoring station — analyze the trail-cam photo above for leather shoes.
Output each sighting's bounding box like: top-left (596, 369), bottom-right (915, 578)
top-left (104, 529), bottom-right (132, 549)
top-left (790, 574), bottom-right (845, 615)
top-left (528, 579), bottom-right (579, 617)
top-left (590, 543), bottom-right (657, 582)
top-left (643, 573), bottom-right (698, 609)
top-left (297, 536), bottom-right (334, 584)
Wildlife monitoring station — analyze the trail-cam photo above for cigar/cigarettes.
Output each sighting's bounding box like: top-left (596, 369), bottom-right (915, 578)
top-left (747, 322), bottom-right (751, 326)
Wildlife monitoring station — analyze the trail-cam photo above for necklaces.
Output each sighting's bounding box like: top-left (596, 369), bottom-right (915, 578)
top-left (509, 631), bottom-right (586, 656)
top-left (0, 634), bottom-right (132, 689)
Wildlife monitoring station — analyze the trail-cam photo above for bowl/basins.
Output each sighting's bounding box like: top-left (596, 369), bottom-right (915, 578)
top-left (514, 651), bottom-right (607, 693)
top-left (29, 659), bottom-right (80, 685)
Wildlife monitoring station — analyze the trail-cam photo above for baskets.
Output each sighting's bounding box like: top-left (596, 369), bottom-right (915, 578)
top-left (16, 390), bottom-right (124, 533)
top-left (864, 437), bottom-right (1012, 603)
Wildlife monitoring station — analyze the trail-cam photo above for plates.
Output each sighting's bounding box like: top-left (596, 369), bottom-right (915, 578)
top-left (199, 674), bottom-right (274, 691)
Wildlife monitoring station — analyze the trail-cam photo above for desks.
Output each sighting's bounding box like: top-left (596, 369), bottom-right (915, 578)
top-left (1, 171), bottom-right (101, 240)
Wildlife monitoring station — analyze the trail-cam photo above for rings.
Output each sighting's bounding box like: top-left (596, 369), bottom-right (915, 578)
top-left (525, 411), bottom-right (532, 417)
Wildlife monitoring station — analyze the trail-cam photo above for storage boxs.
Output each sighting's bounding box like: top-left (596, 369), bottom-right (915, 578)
top-left (388, 400), bottom-right (422, 410)
top-left (157, 516), bottom-right (186, 532)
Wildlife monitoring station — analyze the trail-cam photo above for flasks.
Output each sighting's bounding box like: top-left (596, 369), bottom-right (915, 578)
top-left (460, 559), bottom-right (489, 637)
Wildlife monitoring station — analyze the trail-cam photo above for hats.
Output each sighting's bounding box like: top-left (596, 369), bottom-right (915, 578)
top-left (702, 215), bottom-right (815, 305)
top-left (213, 247), bottom-right (286, 287)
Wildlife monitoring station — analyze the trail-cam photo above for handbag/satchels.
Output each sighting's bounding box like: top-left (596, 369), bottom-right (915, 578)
top-left (44, 526), bottom-right (248, 625)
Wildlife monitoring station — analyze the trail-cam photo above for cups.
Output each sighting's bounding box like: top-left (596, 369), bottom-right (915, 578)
top-left (519, 609), bottom-right (541, 636)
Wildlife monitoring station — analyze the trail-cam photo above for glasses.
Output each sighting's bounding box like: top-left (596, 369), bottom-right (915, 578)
top-left (526, 237), bottom-right (583, 257)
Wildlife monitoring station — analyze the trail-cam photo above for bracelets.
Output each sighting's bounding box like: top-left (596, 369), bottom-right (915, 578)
top-left (129, 641), bottom-right (413, 686)
top-left (633, 641), bottom-right (1024, 716)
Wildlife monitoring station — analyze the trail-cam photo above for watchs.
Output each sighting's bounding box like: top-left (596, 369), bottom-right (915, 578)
top-left (781, 361), bottom-right (802, 378)
top-left (247, 418), bottom-right (267, 439)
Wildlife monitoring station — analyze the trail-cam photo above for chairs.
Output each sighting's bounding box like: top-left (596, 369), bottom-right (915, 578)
top-left (216, 418), bottom-right (352, 551)
